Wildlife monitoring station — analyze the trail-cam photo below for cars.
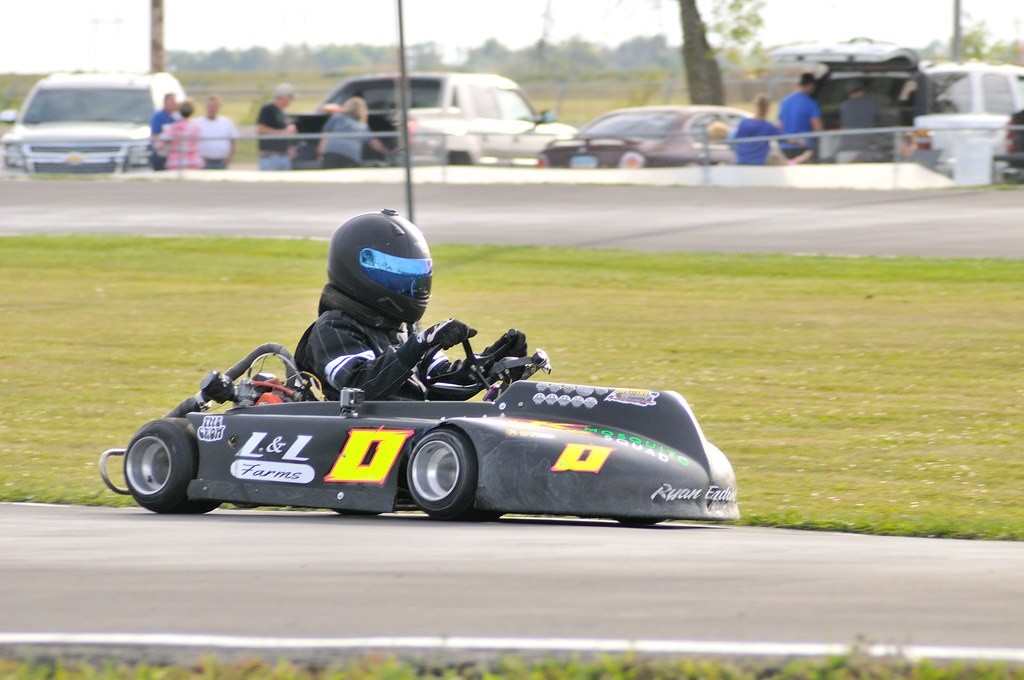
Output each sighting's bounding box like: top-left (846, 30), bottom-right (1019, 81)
top-left (536, 106), bottom-right (764, 169)
top-left (1, 73), bottom-right (191, 172)
top-left (282, 71), bottom-right (578, 165)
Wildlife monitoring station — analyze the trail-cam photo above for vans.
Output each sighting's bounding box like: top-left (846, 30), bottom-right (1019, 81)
top-left (777, 39), bottom-right (1022, 182)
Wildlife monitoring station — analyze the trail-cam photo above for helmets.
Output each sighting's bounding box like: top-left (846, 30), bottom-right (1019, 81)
top-left (327, 209), bottom-right (433, 324)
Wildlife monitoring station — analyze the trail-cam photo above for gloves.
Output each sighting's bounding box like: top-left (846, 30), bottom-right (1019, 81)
top-left (484, 328), bottom-right (528, 359)
top-left (418, 317), bottom-right (478, 350)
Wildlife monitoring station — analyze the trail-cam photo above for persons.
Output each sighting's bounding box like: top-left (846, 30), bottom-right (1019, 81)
top-left (153, 100), bottom-right (206, 171)
top-left (314, 96), bottom-right (389, 169)
top-left (254, 83), bottom-right (300, 171)
top-left (726, 95), bottom-right (808, 167)
top-left (146, 93), bottom-right (179, 170)
top-left (835, 82), bottom-right (899, 153)
top-left (291, 208), bottom-right (528, 404)
top-left (196, 96), bottom-right (237, 171)
top-left (774, 74), bottom-right (825, 164)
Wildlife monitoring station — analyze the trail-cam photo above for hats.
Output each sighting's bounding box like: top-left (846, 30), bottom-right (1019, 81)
top-left (273, 82), bottom-right (300, 97)
